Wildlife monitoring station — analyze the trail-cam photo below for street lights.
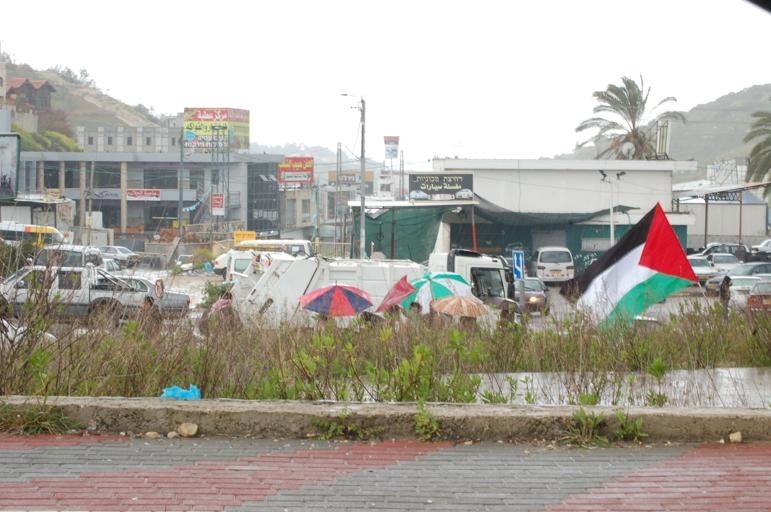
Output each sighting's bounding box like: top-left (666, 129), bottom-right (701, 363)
top-left (339, 93), bottom-right (366, 257)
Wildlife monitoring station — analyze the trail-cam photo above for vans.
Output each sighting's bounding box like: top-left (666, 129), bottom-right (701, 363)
top-left (212, 239), bottom-right (313, 280)
top-left (530, 247), bottom-right (575, 282)
top-left (27, 244), bottom-right (104, 268)
top-left (0, 221), bottom-right (66, 249)
top-left (226, 252), bottom-right (294, 283)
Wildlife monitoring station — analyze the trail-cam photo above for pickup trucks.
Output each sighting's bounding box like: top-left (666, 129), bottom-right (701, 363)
top-left (0, 266), bottom-right (146, 329)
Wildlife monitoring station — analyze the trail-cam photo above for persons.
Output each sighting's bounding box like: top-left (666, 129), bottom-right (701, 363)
top-left (136, 296), bottom-right (162, 335)
top-left (198, 292), bottom-right (245, 337)
top-left (719, 277), bottom-right (733, 325)
top-left (310, 301), bottom-right (520, 335)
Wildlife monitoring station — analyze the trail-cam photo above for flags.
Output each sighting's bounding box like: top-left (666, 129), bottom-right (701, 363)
top-left (558, 201), bottom-right (697, 340)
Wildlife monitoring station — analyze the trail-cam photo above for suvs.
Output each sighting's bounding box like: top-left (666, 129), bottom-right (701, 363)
top-left (688, 243), bottom-right (750, 257)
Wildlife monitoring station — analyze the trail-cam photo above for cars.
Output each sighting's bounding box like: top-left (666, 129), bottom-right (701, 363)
top-left (706, 261), bottom-right (771, 293)
top-left (752, 240), bottom-right (770, 254)
top-left (99, 245), bottom-right (139, 267)
top-left (97, 276), bottom-right (189, 320)
top-left (515, 277), bottom-right (550, 316)
top-left (687, 256), bottom-right (720, 282)
top-left (721, 275), bottom-right (761, 297)
top-left (103, 259), bottom-right (123, 274)
top-left (748, 281), bottom-right (771, 309)
top-left (707, 253), bottom-right (741, 273)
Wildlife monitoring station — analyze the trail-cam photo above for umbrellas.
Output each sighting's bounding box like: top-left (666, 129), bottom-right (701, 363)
top-left (298, 282), bottom-right (375, 317)
top-left (430, 295), bottom-right (488, 317)
top-left (399, 271), bottom-right (473, 315)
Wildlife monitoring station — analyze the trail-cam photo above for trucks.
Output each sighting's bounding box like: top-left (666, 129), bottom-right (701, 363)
top-left (230, 249), bottom-right (514, 337)
